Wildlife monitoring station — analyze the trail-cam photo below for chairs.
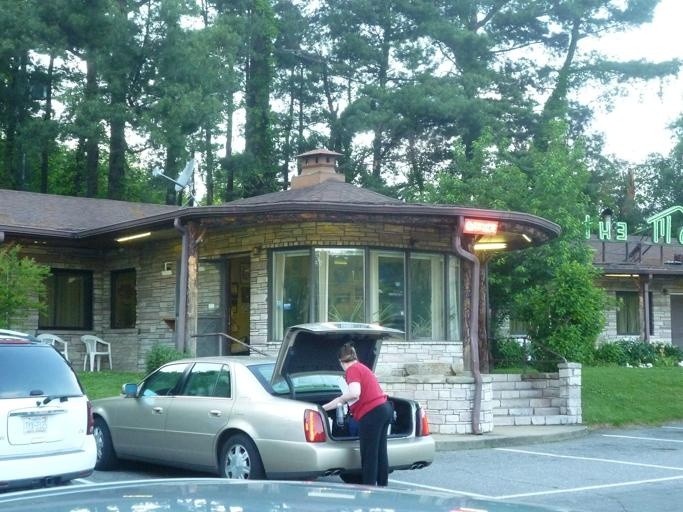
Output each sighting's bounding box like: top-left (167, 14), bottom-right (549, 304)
top-left (37, 334), bottom-right (113, 372)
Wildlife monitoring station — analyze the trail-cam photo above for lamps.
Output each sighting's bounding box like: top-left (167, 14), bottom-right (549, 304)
top-left (161, 261), bottom-right (173, 276)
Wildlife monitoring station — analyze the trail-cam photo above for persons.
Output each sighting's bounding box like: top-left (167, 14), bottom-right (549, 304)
top-left (318, 338), bottom-right (394, 489)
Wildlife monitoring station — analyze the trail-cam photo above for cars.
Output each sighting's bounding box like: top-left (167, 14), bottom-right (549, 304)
top-left (90, 319), bottom-right (437, 481)
top-left (0, 327), bottom-right (100, 493)
top-left (0, 477), bottom-right (569, 511)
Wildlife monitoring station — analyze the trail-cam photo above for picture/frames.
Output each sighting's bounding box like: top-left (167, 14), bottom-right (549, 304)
top-left (230, 263), bottom-right (249, 314)
